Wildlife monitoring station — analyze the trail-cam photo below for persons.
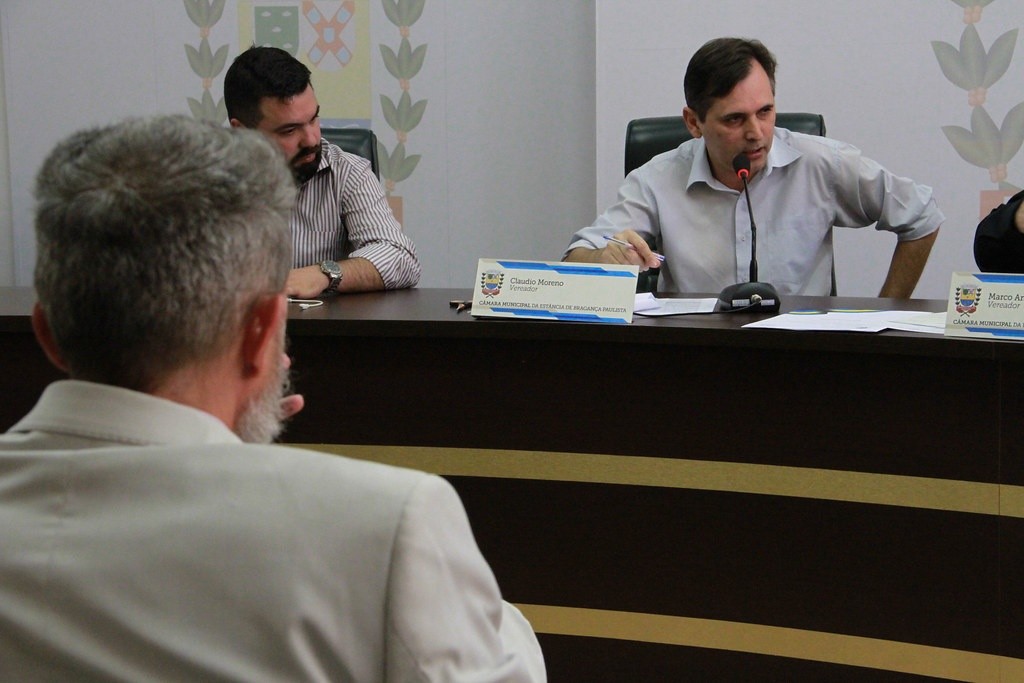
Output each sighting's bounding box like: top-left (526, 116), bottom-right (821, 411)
top-left (0, 108), bottom-right (547, 683)
top-left (224, 44), bottom-right (422, 299)
top-left (561, 37), bottom-right (945, 299)
top-left (973, 189), bottom-right (1024, 275)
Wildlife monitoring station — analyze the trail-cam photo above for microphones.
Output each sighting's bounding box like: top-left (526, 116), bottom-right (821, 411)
top-left (712, 153), bottom-right (780, 314)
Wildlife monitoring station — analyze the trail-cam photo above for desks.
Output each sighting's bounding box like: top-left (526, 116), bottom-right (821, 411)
top-left (0, 284), bottom-right (1024, 683)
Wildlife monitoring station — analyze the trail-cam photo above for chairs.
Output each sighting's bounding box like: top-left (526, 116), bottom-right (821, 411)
top-left (624, 112), bottom-right (838, 297)
top-left (321, 127), bottom-right (381, 183)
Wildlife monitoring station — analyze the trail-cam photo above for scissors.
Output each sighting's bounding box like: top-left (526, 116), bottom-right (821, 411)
top-left (450, 298), bottom-right (472, 311)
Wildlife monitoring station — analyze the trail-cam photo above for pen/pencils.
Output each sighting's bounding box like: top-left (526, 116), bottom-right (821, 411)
top-left (603, 234), bottom-right (666, 262)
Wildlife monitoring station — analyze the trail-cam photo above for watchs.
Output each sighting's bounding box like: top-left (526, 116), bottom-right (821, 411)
top-left (316, 260), bottom-right (343, 295)
